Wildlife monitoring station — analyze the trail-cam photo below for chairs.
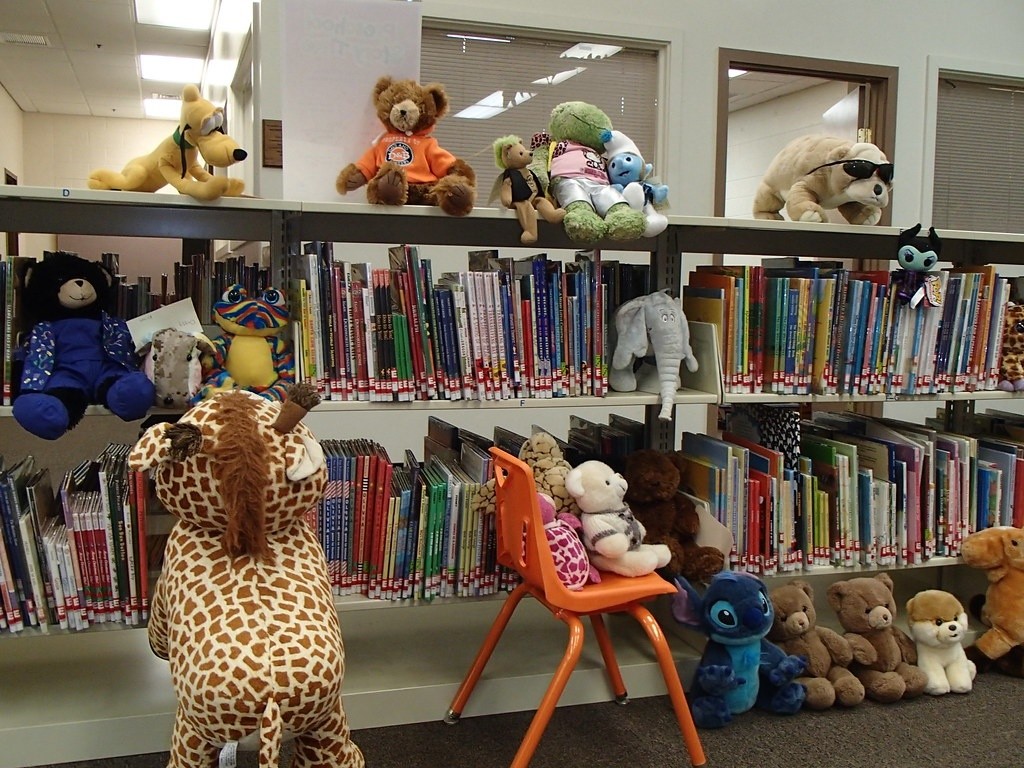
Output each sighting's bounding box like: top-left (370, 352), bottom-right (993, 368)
top-left (444, 446), bottom-right (707, 768)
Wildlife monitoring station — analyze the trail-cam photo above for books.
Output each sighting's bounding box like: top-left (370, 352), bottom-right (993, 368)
top-left (303, 412), bottom-right (645, 603)
top-left (0, 444), bottom-right (161, 637)
top-left (291, 242), bottom-right (650, 402)
top-left (670, 402), bottom-right (1024, 576)
top-left (0, 251), bottom-right (271, 406)
top-left (683, 258), bottom-right (1011, 395)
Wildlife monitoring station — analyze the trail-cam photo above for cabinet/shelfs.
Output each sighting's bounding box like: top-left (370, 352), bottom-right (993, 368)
top-left (0, 184), bottom-right (1024, 768)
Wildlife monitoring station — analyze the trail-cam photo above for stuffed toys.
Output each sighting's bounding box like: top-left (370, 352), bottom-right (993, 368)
top-left (128, 381), bottom-right (365, 768)
top-left (894, 223), bottom-right (943, 310)
top-left (12, 252), bottom-right (157, 440)
top-left (763, 525), bottom-right (1024, 710)
top-left (336, 73), bottom-right (669, 244)
top-left (673, 570), bottom-right (806, 728)
top-left (140, 327), bottom-right (217, 409)
top-left (996, 301), bottom-right (1024, 392)
top-left (88, 83), bottom-right (249, 199)
top-left (752, 134), bottom-right (896, 225)
top-left (608, 288), bottom-right (698, 422)
top-left (191, 283), bottom-right (295, 405)
top-left (472, 429), bottom-right (725, 591)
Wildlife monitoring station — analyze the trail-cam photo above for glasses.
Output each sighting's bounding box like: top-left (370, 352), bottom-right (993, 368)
top-left (807, 159), bottom-right (894, 185)
